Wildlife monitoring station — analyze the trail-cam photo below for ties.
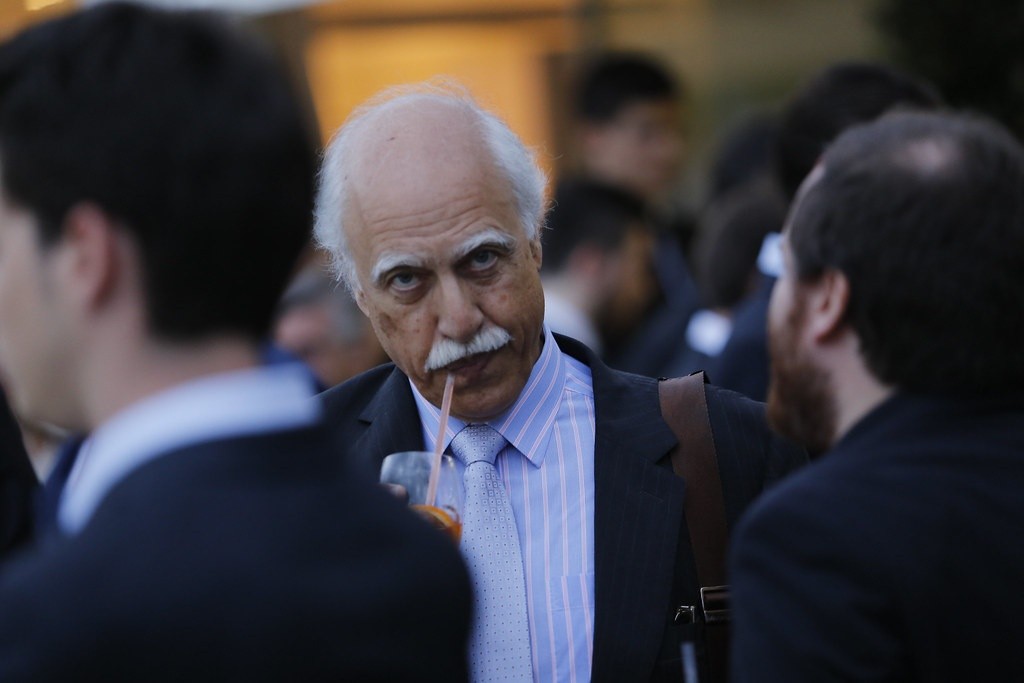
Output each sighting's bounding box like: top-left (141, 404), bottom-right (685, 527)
top-left (447, 419), bottom-right (535, 683)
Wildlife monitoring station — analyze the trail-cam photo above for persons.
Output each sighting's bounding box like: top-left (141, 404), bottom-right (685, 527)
top-left (0, 0), bottom-right (479, 683)
top-left (526, 39), bottom-right (945, 388)
top-left (285, 81), bottom-right (810, 682)
top-left (275, 265), bottom-right (390, 388)
top-left (722, 107), bottom-right (1024, 683)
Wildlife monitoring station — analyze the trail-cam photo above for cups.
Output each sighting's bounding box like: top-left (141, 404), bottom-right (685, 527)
top-left (380, 452), bottom-right (462, 544)
